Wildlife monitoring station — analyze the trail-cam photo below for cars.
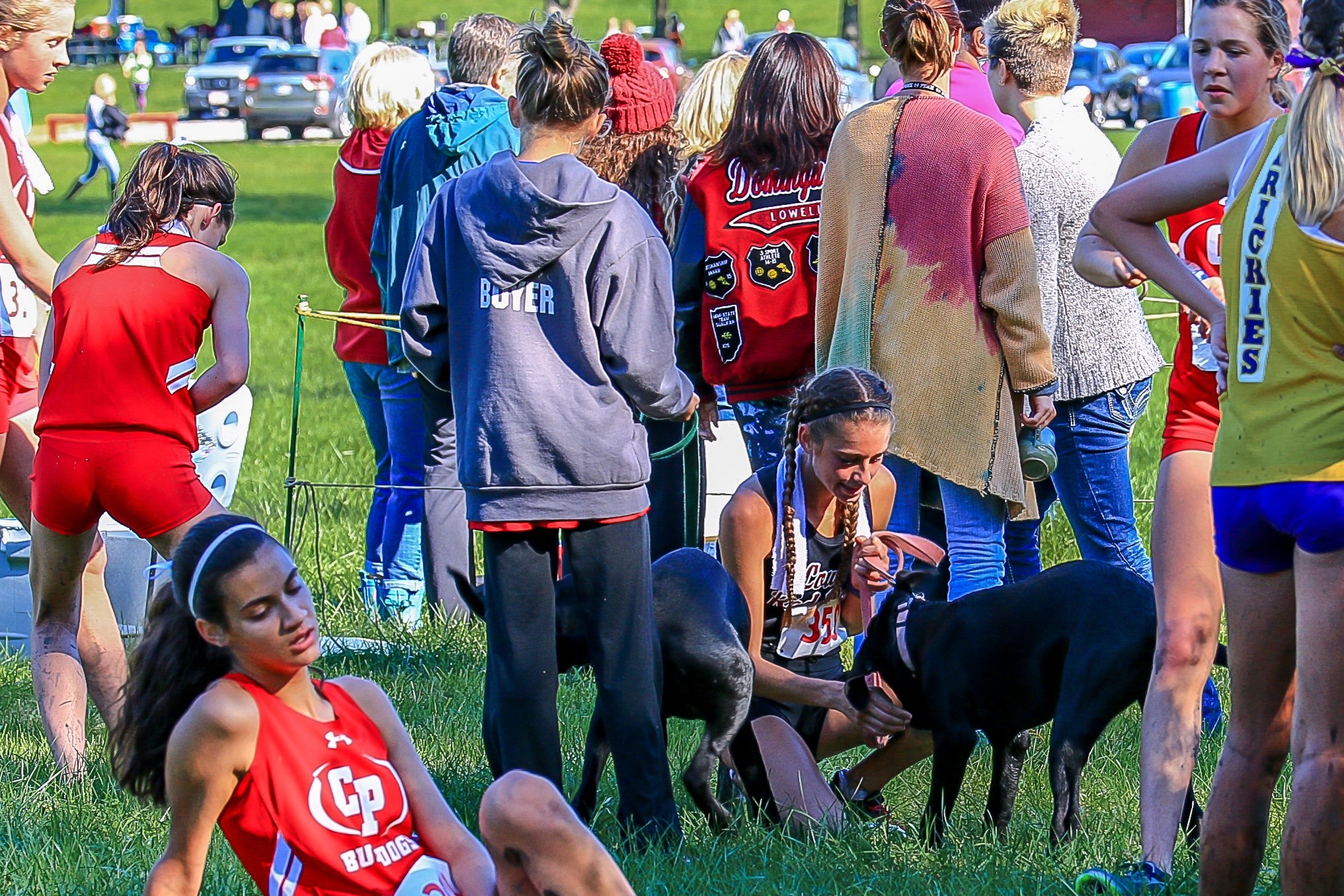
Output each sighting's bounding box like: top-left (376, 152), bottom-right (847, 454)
top-left (244, 51), bottom-right (360, 140)
top-left (1120, 41), bottom-right (1201, 123)
top-left (180, 35), bottom-right (292, 118)
top-left (1066, 42), bottom-right (1144, 131)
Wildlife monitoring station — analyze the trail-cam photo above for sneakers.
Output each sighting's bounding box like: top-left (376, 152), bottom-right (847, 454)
top-left (1075, 861), bottom-right (1173, 896)
top-left (827, 769), bottom-right (907, 841)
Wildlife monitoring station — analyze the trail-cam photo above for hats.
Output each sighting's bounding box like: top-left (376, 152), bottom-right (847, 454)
top-left (598, 33), bottom-right (676, 135)
top-left (954, 0), bottom-right (1003, 31)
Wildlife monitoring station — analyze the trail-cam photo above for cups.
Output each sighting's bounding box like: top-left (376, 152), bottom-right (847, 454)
top-left (1018, 424), bottom-right (1059, 481)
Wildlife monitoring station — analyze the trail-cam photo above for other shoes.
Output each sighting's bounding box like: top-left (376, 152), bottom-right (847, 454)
top-left (1200, 675), bottom-right (1224, 740)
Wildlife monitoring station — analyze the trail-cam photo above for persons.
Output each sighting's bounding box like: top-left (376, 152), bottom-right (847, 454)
top-left (108, 513), bottom-right (640, 896)
top-left (0, 0), bottom-right (1344, 896)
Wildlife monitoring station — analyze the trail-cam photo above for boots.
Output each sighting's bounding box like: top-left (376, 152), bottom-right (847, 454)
top-left (61, 181), bottom-right (83, 201)
top-left (358, 567), bottom-right (386, 623)
top-left (110, 183), bottom-right (118, 204)
top-left (379, 578), bottom-right (425, 631)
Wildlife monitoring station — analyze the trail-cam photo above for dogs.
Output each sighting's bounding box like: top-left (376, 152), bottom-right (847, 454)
top-left (842, 569), bottom-right (1229, 868)
top-left (445, 548), bottom-right (754, 843)
